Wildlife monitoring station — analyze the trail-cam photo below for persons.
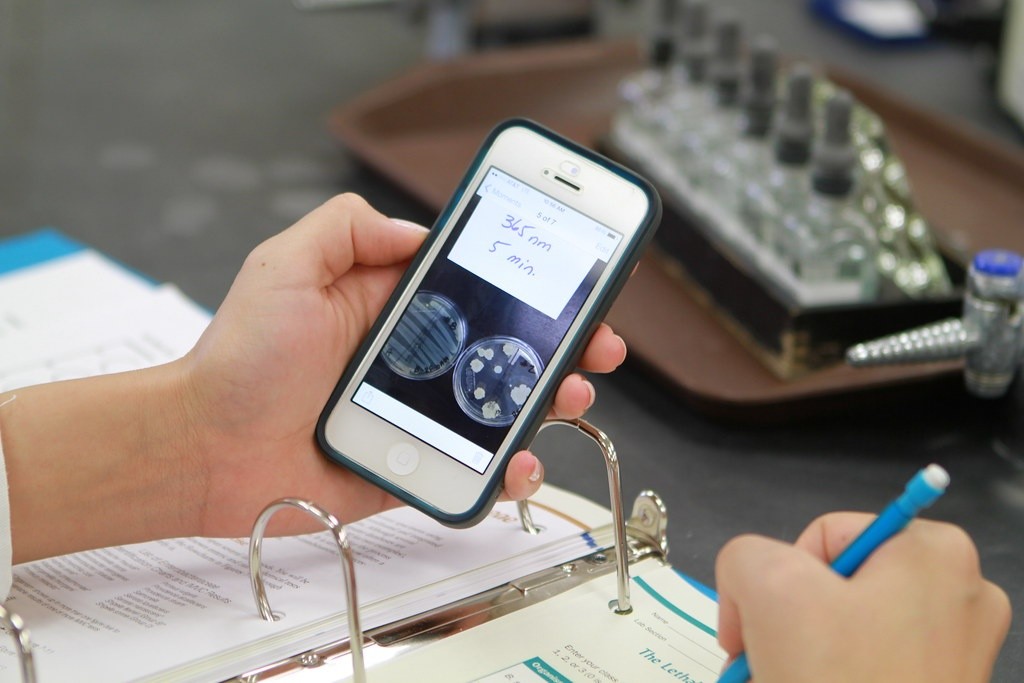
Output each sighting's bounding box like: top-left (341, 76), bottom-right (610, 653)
top-left (0, 194), bottom-right (1011, 683)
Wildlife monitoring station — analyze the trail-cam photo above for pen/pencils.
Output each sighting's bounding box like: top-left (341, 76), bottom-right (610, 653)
top-left (715, 463), bottom-right (951, 683)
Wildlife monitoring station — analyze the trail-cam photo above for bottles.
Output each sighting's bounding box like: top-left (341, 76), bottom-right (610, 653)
top-left (964, 249), bottom-right (1024, 397)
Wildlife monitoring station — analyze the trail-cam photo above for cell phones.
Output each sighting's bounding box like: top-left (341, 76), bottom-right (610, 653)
top-left (314, 116), bottom-right (661, 528)
